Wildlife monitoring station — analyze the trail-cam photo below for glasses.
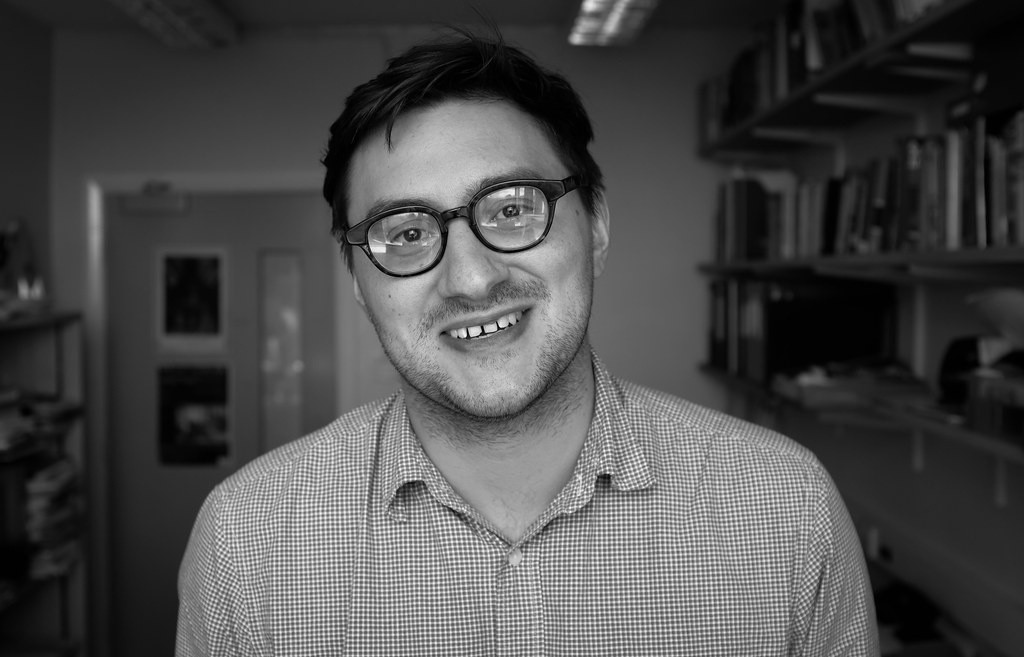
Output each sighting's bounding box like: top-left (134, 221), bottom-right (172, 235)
top-left (341, 175), bottom-right (584, 279)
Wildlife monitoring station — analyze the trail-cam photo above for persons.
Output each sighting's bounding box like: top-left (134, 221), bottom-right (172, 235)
top-left (175, 35), bottom-right (881, 657)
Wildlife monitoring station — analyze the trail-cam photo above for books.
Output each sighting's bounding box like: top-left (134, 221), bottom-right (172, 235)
top-left (695, 0), bottom-right (1024, 392)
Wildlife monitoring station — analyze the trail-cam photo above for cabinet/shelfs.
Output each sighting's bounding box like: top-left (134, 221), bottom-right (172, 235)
top-left (0, 308), bottom-right (93, 657)
top-left (702, 0), bottom-right (1024, 494)
